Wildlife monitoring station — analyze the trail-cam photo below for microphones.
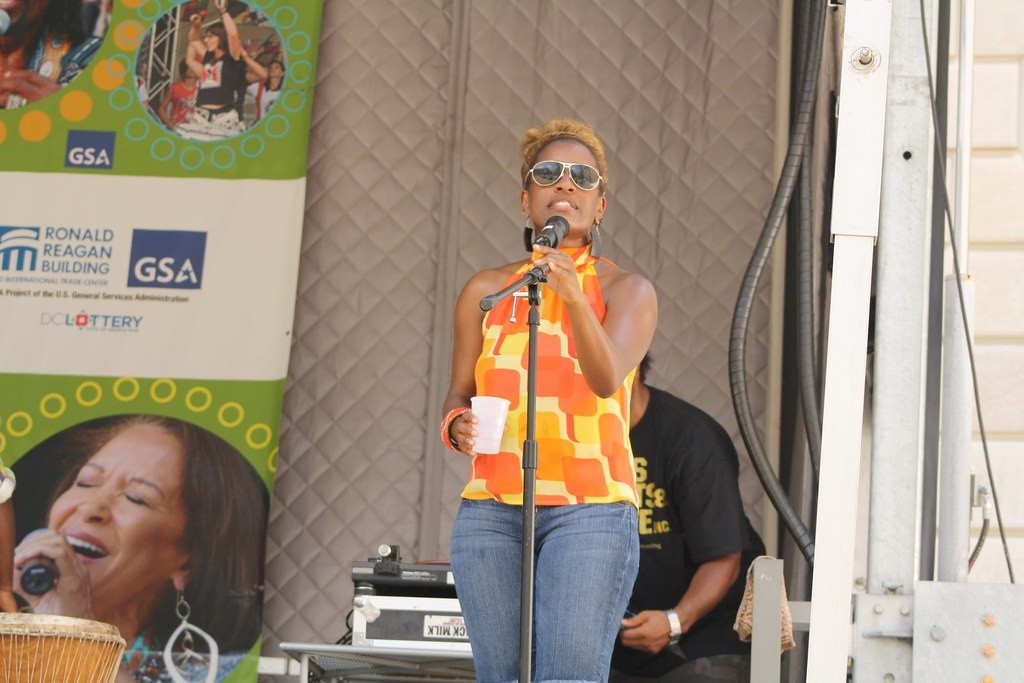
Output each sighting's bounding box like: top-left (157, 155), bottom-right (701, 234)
top-left (20, 527), bottom-right (57, 595)
top-left (534, 215), bottom-right (571, 247)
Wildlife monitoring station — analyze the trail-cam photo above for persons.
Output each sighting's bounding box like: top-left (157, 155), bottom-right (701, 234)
top-left (135, 0), bottom-right (285, 127)
top-left (1, 0), bottom-right (104, 109)
top-left (606, 347), bottom-right (746, 683)
top-left (1, 411), bottom-right (267, 678)
top-left (438, 119), bottom-right (657, 683)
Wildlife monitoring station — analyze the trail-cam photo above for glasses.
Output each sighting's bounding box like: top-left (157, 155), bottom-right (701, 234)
top-left (524, 159), bottom-right (604, 192)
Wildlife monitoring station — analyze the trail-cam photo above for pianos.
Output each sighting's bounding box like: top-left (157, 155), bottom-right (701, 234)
top-left (351, 543), bottom-right (455, 599)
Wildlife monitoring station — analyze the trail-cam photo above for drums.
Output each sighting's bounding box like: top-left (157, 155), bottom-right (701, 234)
top-left (0, 611), bottom-right (127, 683)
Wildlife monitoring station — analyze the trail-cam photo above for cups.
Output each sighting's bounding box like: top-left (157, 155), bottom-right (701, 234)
top-left (469, 395), bottom-right (512, 455)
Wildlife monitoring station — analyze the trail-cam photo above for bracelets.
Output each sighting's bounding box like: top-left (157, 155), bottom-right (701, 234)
top-left (440, 407), bottom-right (471, 454)
top-left (219, 9), bottom-right (228, 15)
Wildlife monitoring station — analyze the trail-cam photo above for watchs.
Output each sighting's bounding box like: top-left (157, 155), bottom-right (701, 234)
top-left (666, 610), bottom-right (682, 645)
